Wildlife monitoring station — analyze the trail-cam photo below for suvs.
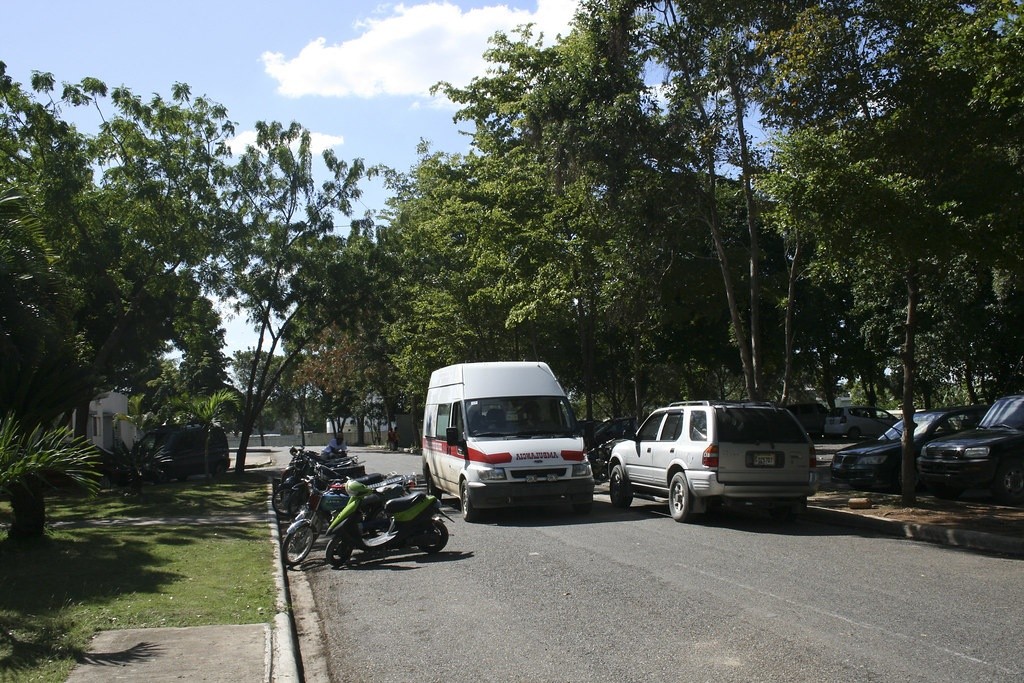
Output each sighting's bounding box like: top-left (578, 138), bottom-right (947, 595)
top-left (609, 400), bottom-right (821, 525)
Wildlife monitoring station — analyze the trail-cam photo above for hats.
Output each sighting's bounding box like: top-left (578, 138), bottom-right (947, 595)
top-left (336, 433), bottom-right (343, 439)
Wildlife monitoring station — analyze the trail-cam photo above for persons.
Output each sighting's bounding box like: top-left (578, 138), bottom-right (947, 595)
top-left (388, 426), bottom-right (400, 451)
top-left (519, 402), bottom-right (561, 431)
top-left (321, 433), bottom-right (347, 460)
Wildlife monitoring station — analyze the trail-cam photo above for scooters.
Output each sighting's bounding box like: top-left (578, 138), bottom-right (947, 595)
top-left (320, 475), bottom-right (456, 568)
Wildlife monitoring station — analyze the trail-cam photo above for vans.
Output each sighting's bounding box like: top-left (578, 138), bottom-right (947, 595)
top-left (422, 361), bottom-right (596, 521)
top-left (128, 423), bottom-right (231, 489)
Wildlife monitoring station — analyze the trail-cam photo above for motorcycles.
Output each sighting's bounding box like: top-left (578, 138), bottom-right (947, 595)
top-left (271, 444), bottom-right (421, 565)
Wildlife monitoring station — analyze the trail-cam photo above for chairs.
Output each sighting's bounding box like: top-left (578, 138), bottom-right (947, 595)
top-left (486, 409), bottom-right (506, 430)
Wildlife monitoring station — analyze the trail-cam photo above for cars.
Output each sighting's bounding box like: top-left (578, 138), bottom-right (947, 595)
top-left (50, 439), bottom-right (145, 489)
top-left (563, 416), bottom-right (653, 480)
top-left (915, 394), bottom-right (1024, 505)
top-left (785, 400), bottom-right (829, 434)
top-left (828, 405), bottom-right (991, 491)
top-left (824, 405), bottom-right (901, 439)
